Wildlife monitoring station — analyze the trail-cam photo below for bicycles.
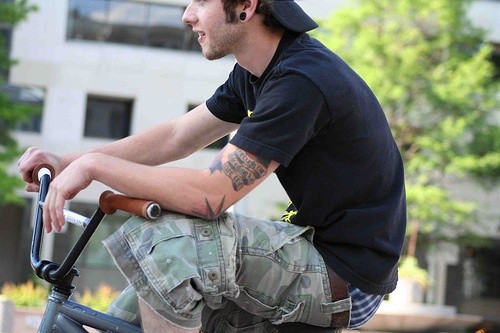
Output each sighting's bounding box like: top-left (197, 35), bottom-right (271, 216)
top-left (30, 161), bottom-right (161, 333)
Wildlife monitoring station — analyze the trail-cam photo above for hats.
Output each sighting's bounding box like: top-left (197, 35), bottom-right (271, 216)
top-left (271, 0), bottom-right (319, 31)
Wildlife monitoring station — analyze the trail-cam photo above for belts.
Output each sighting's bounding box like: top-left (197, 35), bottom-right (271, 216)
top-left (326, 262), bottom-right (350, 328)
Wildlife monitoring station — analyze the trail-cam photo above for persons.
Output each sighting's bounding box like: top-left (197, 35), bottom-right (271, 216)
top-left (17, 0), bottom-right (408, 333)
